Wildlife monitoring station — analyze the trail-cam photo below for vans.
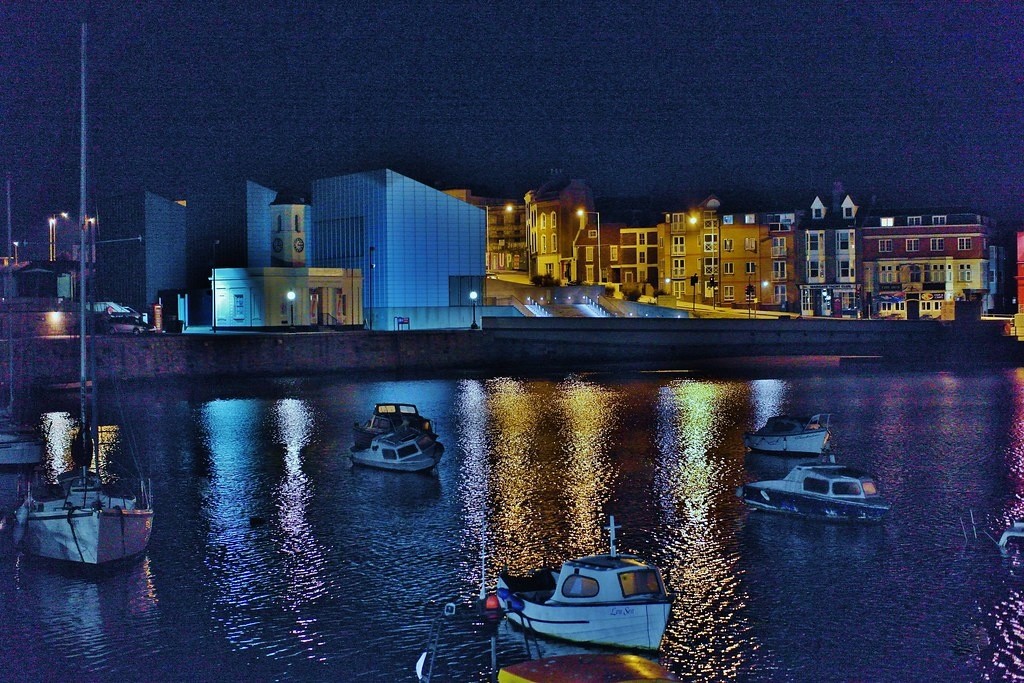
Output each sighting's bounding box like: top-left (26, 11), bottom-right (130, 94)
top-left (99, 312), bottom-right (143, 320)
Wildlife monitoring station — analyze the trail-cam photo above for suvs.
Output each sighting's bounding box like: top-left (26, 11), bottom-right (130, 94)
top-left (107, 317), bottom-right (146, 335)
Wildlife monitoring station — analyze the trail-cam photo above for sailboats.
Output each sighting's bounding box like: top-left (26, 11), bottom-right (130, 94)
top-left (17, 23), bottom-right (155, 574)
top-left (0, 179), bottom-right (44, 470)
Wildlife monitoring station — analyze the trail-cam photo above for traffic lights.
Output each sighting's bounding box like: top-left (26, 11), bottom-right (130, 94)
top-left (745, 285), bottom-right (750, 295)
top-left (710, 277), bottom-right (714, 286)
top-left (751, 285), bottom-right (754, 292)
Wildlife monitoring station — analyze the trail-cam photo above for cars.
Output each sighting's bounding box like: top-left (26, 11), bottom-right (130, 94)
top-left (486, 272), bottom-right (499, 280)
top-left (565, 280), bottom-right (582, 286)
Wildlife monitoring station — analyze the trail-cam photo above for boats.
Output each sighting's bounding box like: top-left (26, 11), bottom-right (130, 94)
top-left (352, 403), bottom-right (439, 446)
top-left (497, 651), bottom-right (684, 683)
top-left (345, 426), bottom-right (444, 472)
top-left (742, 413), bottom-right (833, 455)
top-left (734, 454), bottom-right (892, 523)
top-left (997, 522), bottom-right (1024, 560)
top-left (498, 515), bottom-right (677, 657)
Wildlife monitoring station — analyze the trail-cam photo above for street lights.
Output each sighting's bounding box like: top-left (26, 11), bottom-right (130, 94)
top-left (369, 246), bottom-right (376, 331)
top-left (13, 242), bottom-right (18, 263)
top-left (665, 278), bottom-right (670, 295)
top-left (577, 210), bottom-right (602, 283)
top-left (49, 218), bottom-right (55, 261)
top-left (506, 206), bottom-right (532, 279)
top-left (470, 292), bottom-right (478, 329)
top-left (690, 218), bottom-right (716, 310)
top-left (84, 218), bottom-right (95, 262)
top-left (53, 213), bottom-right (68, 261)
top-left (288, 292), bottom-right (296, 333)
